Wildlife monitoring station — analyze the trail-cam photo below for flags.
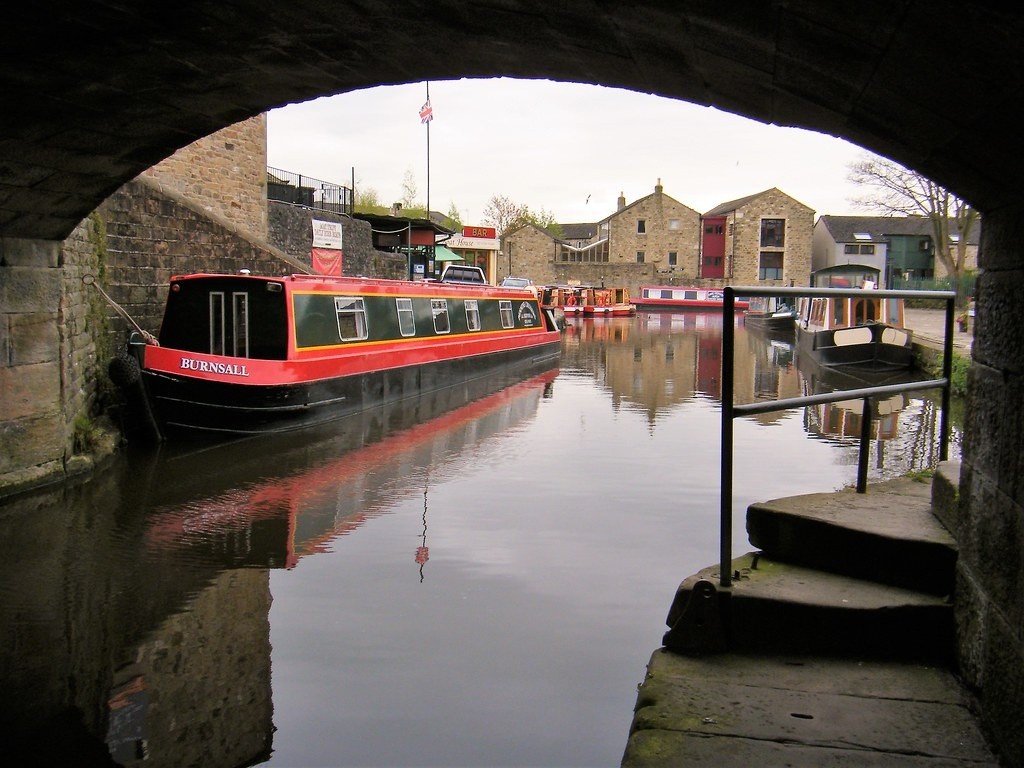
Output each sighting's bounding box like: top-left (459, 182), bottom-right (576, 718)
top-left (419, 101), bottom-right (433, 123)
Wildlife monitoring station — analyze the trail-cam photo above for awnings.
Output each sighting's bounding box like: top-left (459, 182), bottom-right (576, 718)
top-left (417, 245), bottom-right (465, 261)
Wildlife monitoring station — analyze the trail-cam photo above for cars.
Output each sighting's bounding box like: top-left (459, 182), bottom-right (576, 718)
top-left (131, 263), bottom-right (566, 438)
top-left (433, 261), bottom-right (489, 286)
top-left (500, 275), bottom-right (539, 298)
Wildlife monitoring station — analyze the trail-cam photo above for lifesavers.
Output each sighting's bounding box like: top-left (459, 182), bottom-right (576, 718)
top-left (596, 296), bottom-right (606, 308)
top-left (566, 297), bottom-right (577, 306)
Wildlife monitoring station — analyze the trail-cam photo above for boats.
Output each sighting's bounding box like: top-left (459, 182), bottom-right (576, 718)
top-left (119, 340), bottom-right (566, 567)
top-left (540, 284), bottom-right (914, 374)
